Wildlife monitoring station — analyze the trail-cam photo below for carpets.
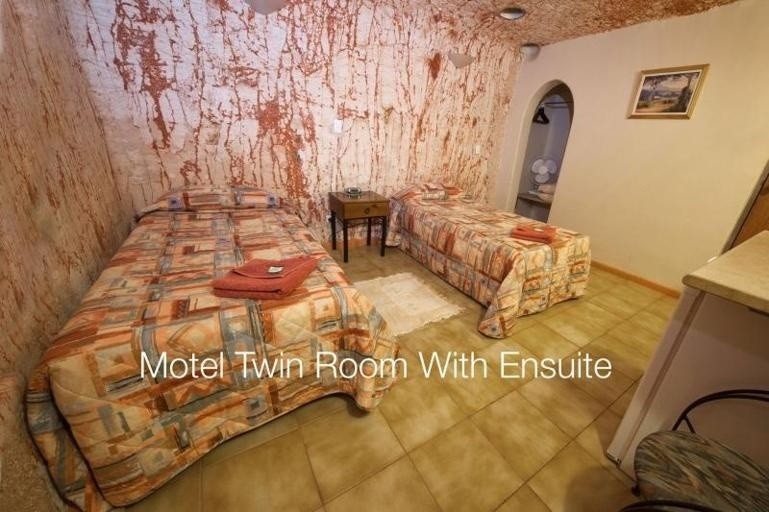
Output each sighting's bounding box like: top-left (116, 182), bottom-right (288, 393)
top-left (353, 271), bottom-right (465, 337)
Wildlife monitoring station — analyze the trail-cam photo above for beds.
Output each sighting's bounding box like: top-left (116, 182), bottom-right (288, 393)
top-left (385, 183), bottom-right (591, 339)
top-left (24, 186), bottom-right (399, 510)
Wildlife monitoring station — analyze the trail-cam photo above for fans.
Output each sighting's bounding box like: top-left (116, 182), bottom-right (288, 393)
top-left (528, 153), bottom-right (559, 195)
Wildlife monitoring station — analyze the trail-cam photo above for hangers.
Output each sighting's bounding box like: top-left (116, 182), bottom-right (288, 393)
top-left (533, 105), bottom-right (549, 124)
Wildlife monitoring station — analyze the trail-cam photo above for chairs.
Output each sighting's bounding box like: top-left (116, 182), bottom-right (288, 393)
top-left (615, 389), bottom-right (769, 511)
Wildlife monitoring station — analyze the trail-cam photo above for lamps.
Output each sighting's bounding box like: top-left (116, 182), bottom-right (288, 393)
top-left (449, 17), bottom-right (480, 68)
top-left (519, 44), bottom-right (540, 60)
top-left (497, 8), bottom-right (526, 20)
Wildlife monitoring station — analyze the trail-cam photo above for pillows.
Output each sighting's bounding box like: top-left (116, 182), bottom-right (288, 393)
top-left (392, 183), bottom-right (463, 207)
top-left (141, 188), bottom-right (286, 213)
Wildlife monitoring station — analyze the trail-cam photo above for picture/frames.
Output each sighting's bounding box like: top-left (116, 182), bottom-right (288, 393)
top-left (625, 64), bottom-right (709, 120)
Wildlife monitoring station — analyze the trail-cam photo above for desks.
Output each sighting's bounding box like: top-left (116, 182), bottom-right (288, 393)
top-left (605, 229), bottom-right (769, 482)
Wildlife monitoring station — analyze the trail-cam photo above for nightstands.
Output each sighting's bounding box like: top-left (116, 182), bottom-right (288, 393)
top-left (327, 191), bottom-right (390, 262)
